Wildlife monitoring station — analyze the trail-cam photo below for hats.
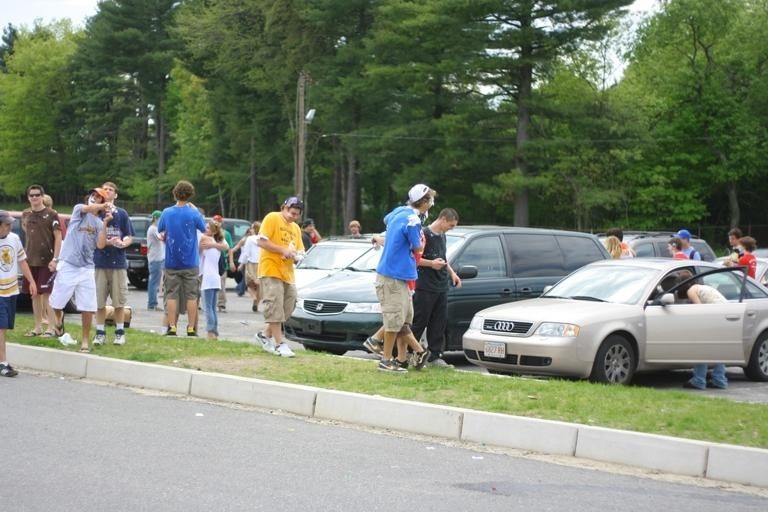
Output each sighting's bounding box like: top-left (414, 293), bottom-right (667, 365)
top-left (214, 215), bottom-right (222, 221)
top-left (0, 209), bottom-right (16, 226)
top-left (303, 218), bottom-right (314, 224)
top-left (670, 230), bottom-right (690, 239)
top-left (405, 184), bottom-right (430, 205)
top-left (89, 187), bottom-right (110, 202)
top-left (285, 197), bottom-right (303, 209)
top-left (152, 211), bottom-right (161, 218)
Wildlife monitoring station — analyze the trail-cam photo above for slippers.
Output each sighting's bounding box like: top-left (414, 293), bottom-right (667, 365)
top-left (40, 330), bottom-right (55, 338)
top-left (55, 310), bottom-right (65, 336)
top-left (24, 330), bottom-right (42, 337)
top-left (79, 346), bottom-right (92, 355)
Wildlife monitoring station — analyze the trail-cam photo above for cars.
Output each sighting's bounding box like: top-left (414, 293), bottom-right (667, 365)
top-left (294, 232), bottom-right (384, 290)
top-left (753, 257), bottom-right (767, 285)
top-left (461, 257), bottom-right (768, 383)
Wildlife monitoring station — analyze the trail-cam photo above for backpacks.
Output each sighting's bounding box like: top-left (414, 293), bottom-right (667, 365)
top-left (690, 249), bottom-right (704, 261)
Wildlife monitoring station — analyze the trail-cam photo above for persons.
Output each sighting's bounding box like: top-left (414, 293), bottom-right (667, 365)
top-left (674, 270), bottom-right (728, 390)
top-left (604, 227), bottom-right (757, 279)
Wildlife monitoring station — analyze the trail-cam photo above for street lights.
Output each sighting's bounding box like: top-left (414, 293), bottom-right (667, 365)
top-left (294, 107), bottom-right (317, 201)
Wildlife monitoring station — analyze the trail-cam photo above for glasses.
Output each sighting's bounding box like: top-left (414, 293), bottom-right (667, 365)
top-left (29, 193), bottom-right (41, 197)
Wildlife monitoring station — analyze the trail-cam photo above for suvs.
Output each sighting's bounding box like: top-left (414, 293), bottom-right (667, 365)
top-left (596, 230), bottom-right (717, 261)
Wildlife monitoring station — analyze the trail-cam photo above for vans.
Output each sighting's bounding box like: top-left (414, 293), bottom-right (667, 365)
top-left (122, 215), bottom-right (254, 290)
top-left (5, 209), bottom-right (80, 313)
top-left (283, 225), bottom-right (611, 356)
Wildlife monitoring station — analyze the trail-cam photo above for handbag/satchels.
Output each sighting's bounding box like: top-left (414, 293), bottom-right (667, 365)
top-left (237, 278), bottom-right (245, 295)
top-left (219, 249), bottom-right (226, 276)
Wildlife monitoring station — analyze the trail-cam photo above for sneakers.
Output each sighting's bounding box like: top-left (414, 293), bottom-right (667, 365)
top-left (252, 299), bottom-right (257, 311)
top-left (683, 380), bottom-right (699, 388)
top-left (706, 379), bottom-right (720, 388)
top-left (1, 362), bottom-right (18, 377)
top-left (113, 329), bottom-right (126, 346)
top-left (273, 339), bottom-right (296, 358)
top-left (92, 329), bottom-right (107, 345)
top-left (148, 306), bottom-right (164, 311)
top-left (255, 331), bottom-right (275, 353)
top-left (162, 322), bottom-right (199, 339)
top-left (219, 306), bottom-right (227, 313)
top-left (362, 337), bottom-right (454, 374)
